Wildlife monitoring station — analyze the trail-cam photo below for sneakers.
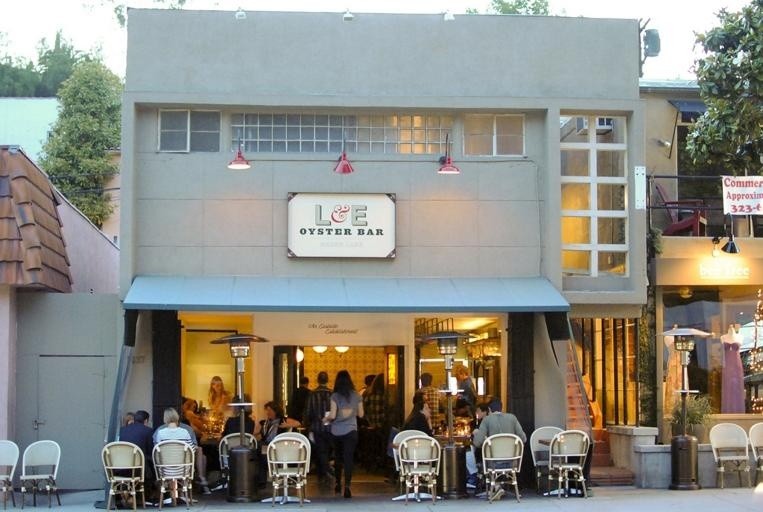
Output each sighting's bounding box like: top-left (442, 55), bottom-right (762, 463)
top-left (335, 485), bottom-right (341, 494)
top-left (492, 487), bottom-right (504, 501)
top-left (344, 488), bottom-right (351, 498)
top-left (195, 476), bottom-right (211, 495)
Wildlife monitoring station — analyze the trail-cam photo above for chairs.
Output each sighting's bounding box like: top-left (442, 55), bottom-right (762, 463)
top-left (481, 426), bottom-right (595, 499)
top-left (100, 431), bottom-right (313, 512)
top-left (0, 436), bottom-right (63, 511)
top-left (655, 182), bottom-right (706, 237)
top-left (390, 428), bottom-right (444, 505)
top-left (708, 423), bottom-right (762, 488)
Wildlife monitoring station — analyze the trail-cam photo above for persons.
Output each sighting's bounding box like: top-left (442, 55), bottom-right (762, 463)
top-left (361, 374), bottom-right (376, 399)
top-left (472, 395), bottom-right (527, 503)
top-left (404, 362), bottom-right (488, 495)
top-left (319, 369), bottom-right (365, 498)
top-left (290, 376), bottom-right (312, 417)
top-left (361, 372), bottom-right (385, 430)
top-left (207, 375), bottom-right (233, 412)
top-left (720, 321), bottom-right (748, 413)
top-left (115, 396), bottom-right (289, 508)
top-left (300, 370), bottom-right (334, 487)
top-left (662, 323), bottom-right (690, 412)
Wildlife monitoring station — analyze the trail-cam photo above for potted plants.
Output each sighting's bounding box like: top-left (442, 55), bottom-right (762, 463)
top-left (666, 394), bottom-right (712, 443)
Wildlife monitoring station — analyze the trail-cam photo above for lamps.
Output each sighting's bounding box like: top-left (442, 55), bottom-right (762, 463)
top-left (331, 137), bottom-right (353, 174)
top-left (435, 132), bottom-right (460, 175)
top-left (712, 211), bottom-right (739, 255)
top-left (225, 136), bottom-right (251, 171)
